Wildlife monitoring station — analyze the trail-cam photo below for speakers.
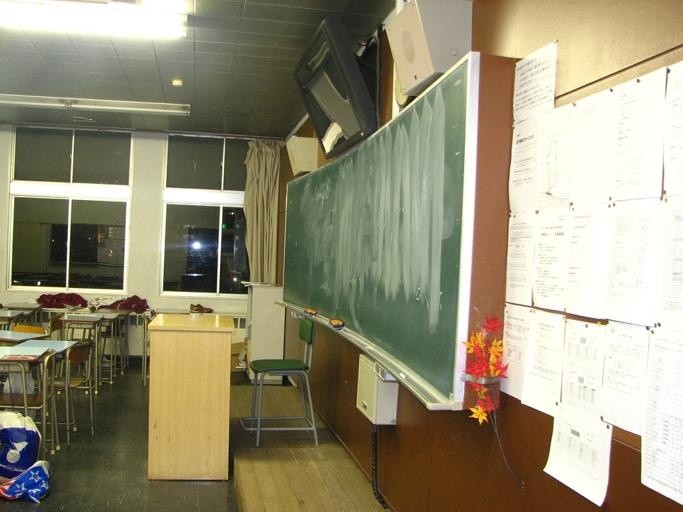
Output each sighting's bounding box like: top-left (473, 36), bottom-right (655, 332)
top-left (384, 0), bottom-right (473, 97)
top-left (285, 135), bottom-right (319, 177)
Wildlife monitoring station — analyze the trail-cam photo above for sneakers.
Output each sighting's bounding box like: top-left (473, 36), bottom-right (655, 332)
top-left (189, 303), bottom-right (213, 313)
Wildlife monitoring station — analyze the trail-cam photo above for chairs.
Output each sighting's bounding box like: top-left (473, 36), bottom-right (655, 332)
top-left (239, 314), bottom-right (324, 447)
top-left (0, 301), bottom-right (149, 469)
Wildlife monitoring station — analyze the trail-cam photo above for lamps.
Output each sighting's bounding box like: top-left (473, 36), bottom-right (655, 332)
top-left (0, 92), bottom-right (194, 119)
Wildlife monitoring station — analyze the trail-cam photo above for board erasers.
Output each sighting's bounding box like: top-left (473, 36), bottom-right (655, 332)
top-left (330, 319), bottom-right (344, 330)
top-left (304, 306), bottom-right (317, 315)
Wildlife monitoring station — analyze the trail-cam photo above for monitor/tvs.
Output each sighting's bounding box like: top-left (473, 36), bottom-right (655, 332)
top-left (291, 14), bottom-right (378, 160)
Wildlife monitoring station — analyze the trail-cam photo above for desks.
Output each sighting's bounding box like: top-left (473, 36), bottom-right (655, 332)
top-left (146, 313), bottom-right (237, 483)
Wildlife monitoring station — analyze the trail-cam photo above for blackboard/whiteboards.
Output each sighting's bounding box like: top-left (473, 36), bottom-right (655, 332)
top-left (274, 50), bottom-right (481, 412)
